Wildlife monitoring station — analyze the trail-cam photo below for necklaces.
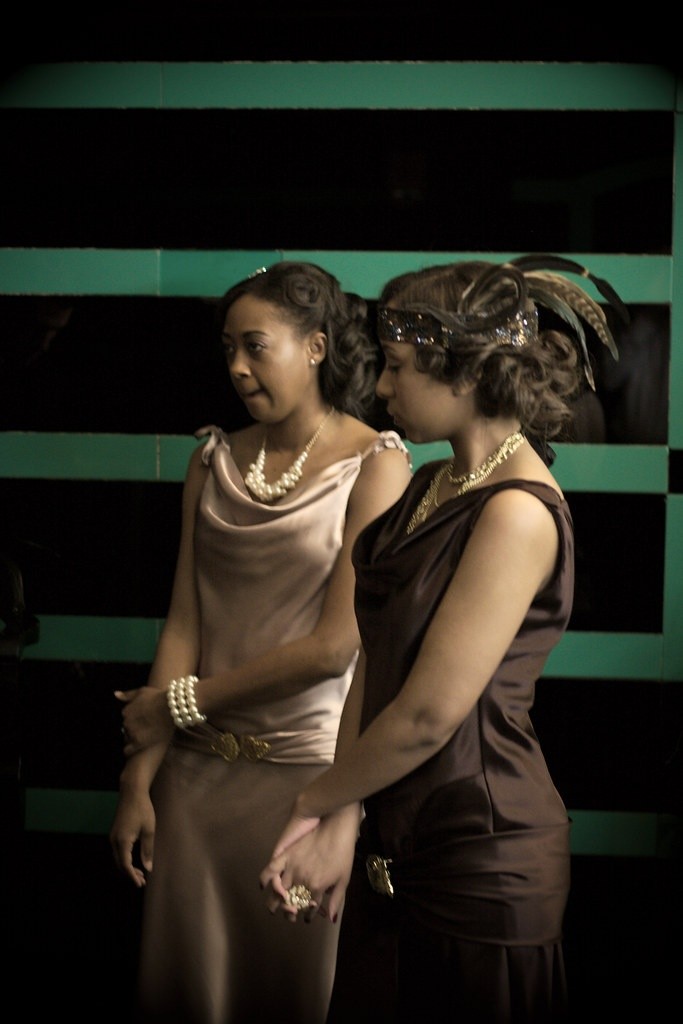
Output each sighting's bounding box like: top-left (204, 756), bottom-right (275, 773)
top-left (407, 428), bottom-right (526, 535)
top-left (246, 405), bottom-right (336, 501)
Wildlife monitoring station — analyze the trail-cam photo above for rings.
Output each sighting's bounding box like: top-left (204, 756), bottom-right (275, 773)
top-left (121, 727), bottom-right (125, 734)
top-left (288, 884), bottom-right (312, 908)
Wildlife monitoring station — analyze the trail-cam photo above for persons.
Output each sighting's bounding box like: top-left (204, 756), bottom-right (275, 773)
top-left (109, 259), bottom-right (413, 1024)
top-left (258, 259), bottom-right (630, 1024)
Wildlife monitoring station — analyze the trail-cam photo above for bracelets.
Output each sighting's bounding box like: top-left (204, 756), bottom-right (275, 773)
top-left (166, 676), bottom-right (207, 730)
